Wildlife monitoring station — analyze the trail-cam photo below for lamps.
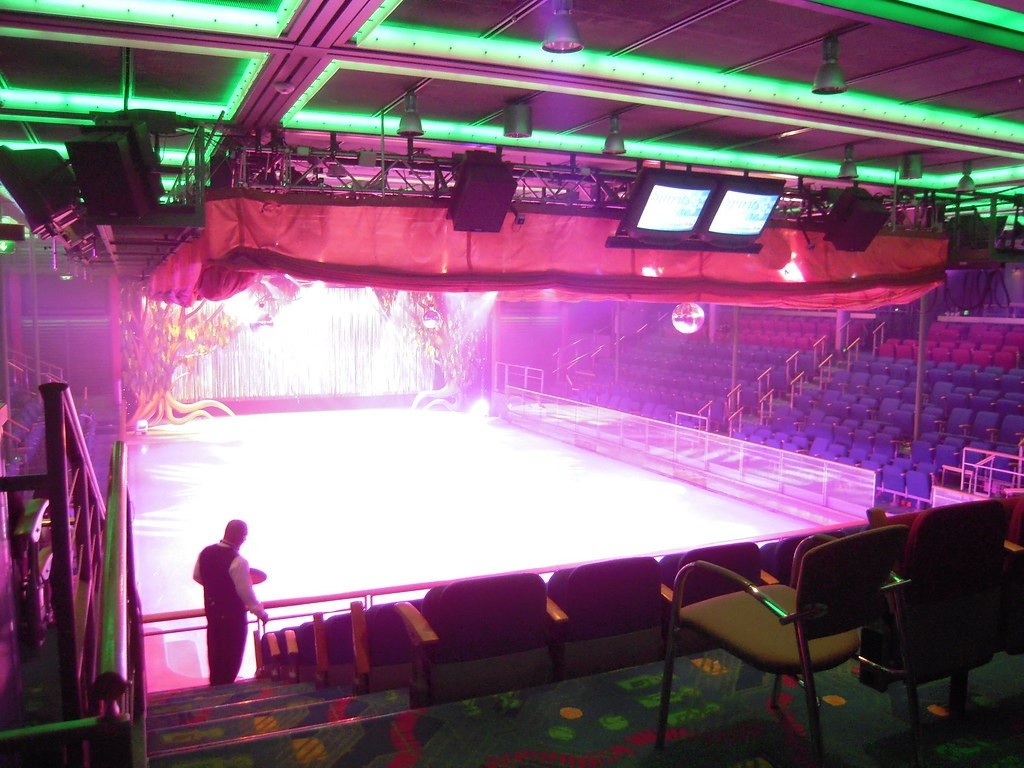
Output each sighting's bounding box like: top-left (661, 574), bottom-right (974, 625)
top-left (898, 154), bottom-right (922, 180)
top-left (0, 146), bottom-right (80, 240)
top-left (504, 104), bottom-right (532, 138)
top-left (396, 89), bottom-right (424, 137)
top-left (64, 119), bottom-right (164, 220)
top-left (811, 31), bottom-right (847, 95)
top-left (837, 144), bottom-right (859, 180)
top-left (540, 0), bottom-right (583, 54)
top-left (447, 146), bottom-right (518, 233)
top-left (603, 114), bottom-right (628, 155)
top-left (821, 182), bottom-right (890, 252)
top-left (954, 160), bottom-right (976, 193)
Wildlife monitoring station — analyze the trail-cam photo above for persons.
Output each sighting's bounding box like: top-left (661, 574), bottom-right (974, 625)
top-left (193, 519), bottom-right (269, 685)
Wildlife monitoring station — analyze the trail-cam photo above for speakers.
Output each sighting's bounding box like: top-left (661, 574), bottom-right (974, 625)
top-left (65, 121), bottom-right (166, 224)
top-left (451, 159), bottom-right (517, 233)
top-left (824, 186), bottom-right (891, 252)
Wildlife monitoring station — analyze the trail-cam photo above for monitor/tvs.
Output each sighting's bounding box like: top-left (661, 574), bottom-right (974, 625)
top-left (616, 171), bottom-right (785, 250)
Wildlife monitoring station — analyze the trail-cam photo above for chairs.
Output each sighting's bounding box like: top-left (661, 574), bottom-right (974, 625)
top-left (0, 379), bottom-right (94, 470)
top-left (559, 313), bottom-right (1024, 512)
top-left (254, 489), bottom-right (1024, 768)
